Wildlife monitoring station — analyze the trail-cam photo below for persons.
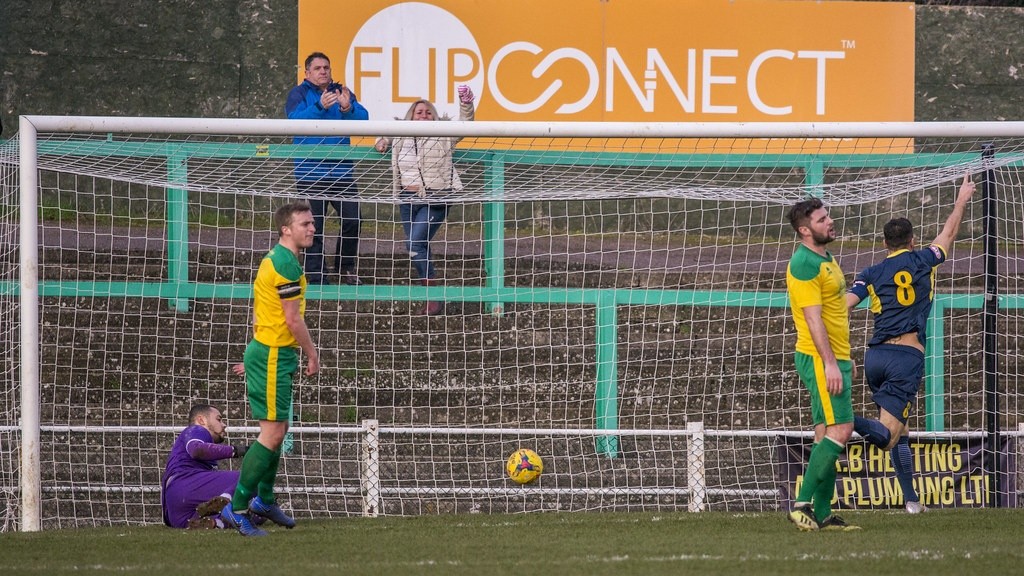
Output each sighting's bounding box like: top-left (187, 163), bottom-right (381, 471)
top-left (785, 199), bottom-right (862, 531)
top-left (221, 203), bottom-right (318, 539)
top-left (285, 52), bottom-right (370, 285)
top-left (845, 170), bottom-right (977, 515)
top-left (162, 404), bottom-right (265, 529)
top-left (373, 85), bottom-right (476, 286)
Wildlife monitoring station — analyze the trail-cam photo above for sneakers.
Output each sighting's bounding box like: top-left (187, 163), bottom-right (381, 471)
top-left (219, 501), bottom-right (267, 539)
top-left (905, 500), bottom-right (933, 515)
top-left (789, 501), bottom-right (861, 533)
top-left (248, 494), bottom-right (296, 530)
top-left (185, 496), bottom-right (229, 530)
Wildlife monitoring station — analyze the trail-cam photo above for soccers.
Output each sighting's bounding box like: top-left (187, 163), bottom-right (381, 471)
top-left (506, 448), bottom-right (544, 485)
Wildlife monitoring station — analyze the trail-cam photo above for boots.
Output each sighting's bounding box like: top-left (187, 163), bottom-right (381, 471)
top-left (423, 278), bottom-right (442, 315)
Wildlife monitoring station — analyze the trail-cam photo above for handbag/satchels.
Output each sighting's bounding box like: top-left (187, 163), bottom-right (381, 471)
top-left (425, 189), bottom-right (454, 208)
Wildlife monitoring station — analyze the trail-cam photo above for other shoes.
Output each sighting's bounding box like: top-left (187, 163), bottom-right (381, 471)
top-left (337, 267), bottom-right (361, 285)
